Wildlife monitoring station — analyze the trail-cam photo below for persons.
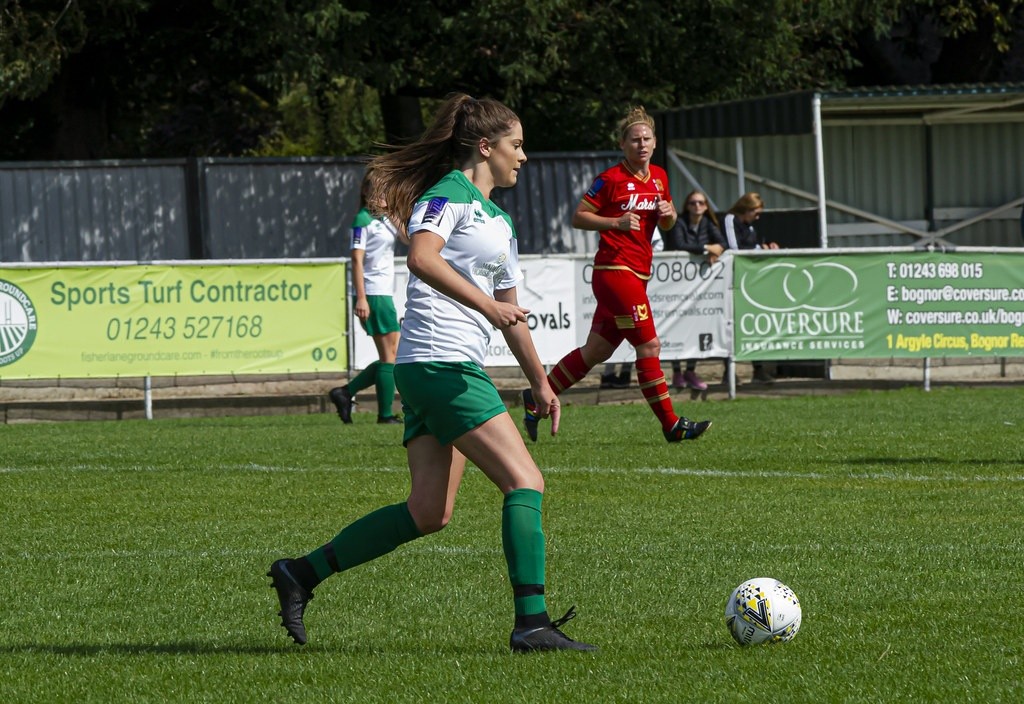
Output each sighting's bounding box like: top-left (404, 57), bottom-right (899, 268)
top-left (518, 106), bottom-right (713, 443)
top-left (722, 191), bottom-right (783, 389)
top-left (263, 93), bottom-right (595, 653)
top-left (659, 188), bottom-right (727, 393)
top-left (329, 164), bottom-right (409, 425)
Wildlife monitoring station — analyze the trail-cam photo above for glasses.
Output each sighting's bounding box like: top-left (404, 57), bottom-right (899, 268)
top-left (689, 201), bottom-right (705, 205)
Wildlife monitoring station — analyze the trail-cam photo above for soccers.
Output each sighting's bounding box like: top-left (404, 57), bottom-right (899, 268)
top-left (724, 578), bottom-right (803, 647)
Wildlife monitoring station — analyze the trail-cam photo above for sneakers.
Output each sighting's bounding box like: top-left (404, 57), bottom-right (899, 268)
top-left (600, 372), bottom-right (631, 387)
top-left (672, 373), bottom-right (687, 387)
top-left (510, 623), bottom-right (599, 656)
top-left (521, 388), bottom-right (542, 442)
top-left (377, 415), bottom-right (404, 424)
top-left (265, 557), bottom-right (309, 646)
top-left (685, 371), bottom-right (708, 390)
top-left (329, 387), bottom-right (354, 425)
top-left (662, 416), bottom-right (713, 443)
